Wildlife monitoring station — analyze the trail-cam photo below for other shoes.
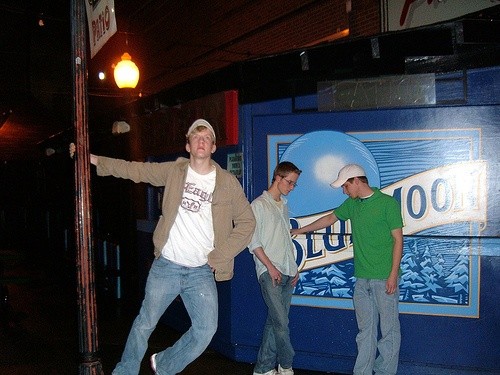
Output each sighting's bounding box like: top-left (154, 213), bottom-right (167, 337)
top-left (253, 368), bottom-right (281, 375)
top-left (151, 353), bottom-right (161, 374)
top-left (277, 363), bottom-right (296, 375)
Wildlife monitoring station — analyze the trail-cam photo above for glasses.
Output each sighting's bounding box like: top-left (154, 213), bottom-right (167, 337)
top-left (279, 175), bottom-right (297, 188)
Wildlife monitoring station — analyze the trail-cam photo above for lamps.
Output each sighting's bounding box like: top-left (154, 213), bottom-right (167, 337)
top-left (114, 51), bottom-right (139, 90)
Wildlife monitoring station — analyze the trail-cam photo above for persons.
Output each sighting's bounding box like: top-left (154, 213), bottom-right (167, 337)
top-left (289, 164), bottom-right (404, 375)
top-left (247, 162), bottom-right (300, 375)
top-left (69, 119), bottom-right (256, 375)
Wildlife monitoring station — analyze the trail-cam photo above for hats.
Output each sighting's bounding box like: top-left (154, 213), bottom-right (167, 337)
top-left (187, 118), bottom-right (215, 139)
top-left (330, 164), bottom-right (365, 189)
